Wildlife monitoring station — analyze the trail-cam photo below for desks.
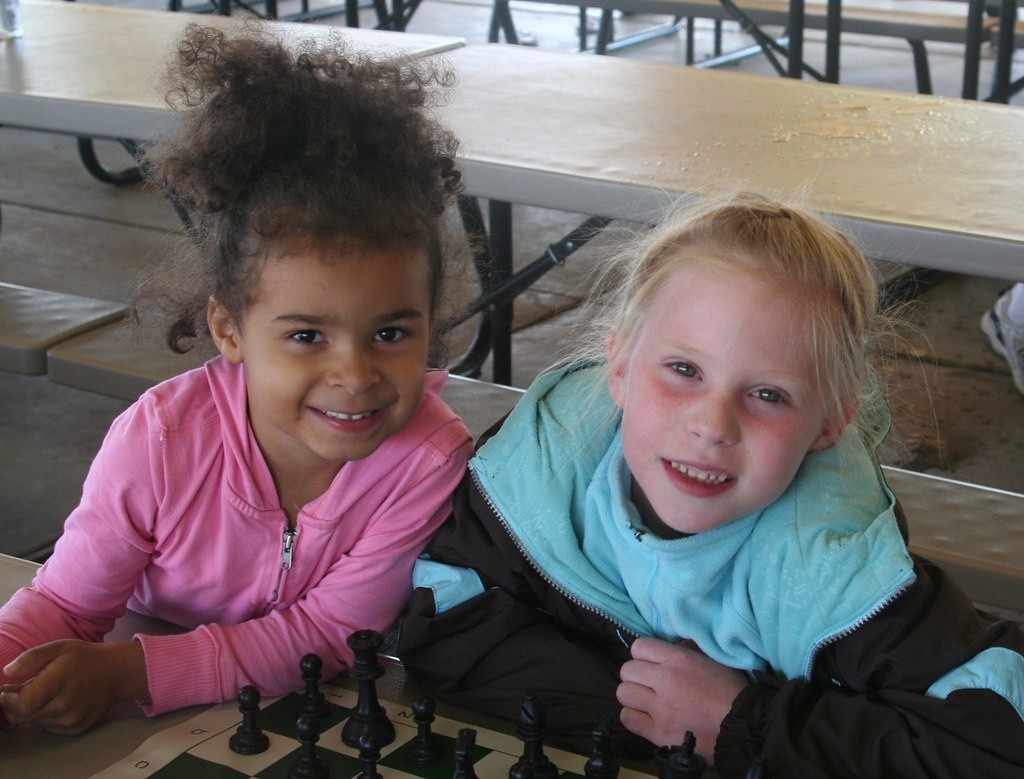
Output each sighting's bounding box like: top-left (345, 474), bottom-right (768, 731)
top-left (0, 0), bottom-right (1024, 779)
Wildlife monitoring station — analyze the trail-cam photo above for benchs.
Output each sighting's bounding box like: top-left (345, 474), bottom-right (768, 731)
top-left (49, 318), bottom-right (1024, 613)
top-left (519, 0), bottom-right (999, 95)
top-left (0, 284), bottom-right (130, 377)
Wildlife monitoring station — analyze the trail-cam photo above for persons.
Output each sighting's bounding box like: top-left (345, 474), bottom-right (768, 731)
top-left (0, 22), bottom-right (475, 736)
top-left (399, 192), bottom-right (1023, 779)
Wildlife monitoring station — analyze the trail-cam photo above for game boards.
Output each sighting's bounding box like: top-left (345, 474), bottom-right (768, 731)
top-left (84, 682), bottom-right (660, 779)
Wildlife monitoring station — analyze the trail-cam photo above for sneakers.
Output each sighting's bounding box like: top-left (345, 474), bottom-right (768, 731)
top-left (979, 291), bottom-right (1024, 400)
